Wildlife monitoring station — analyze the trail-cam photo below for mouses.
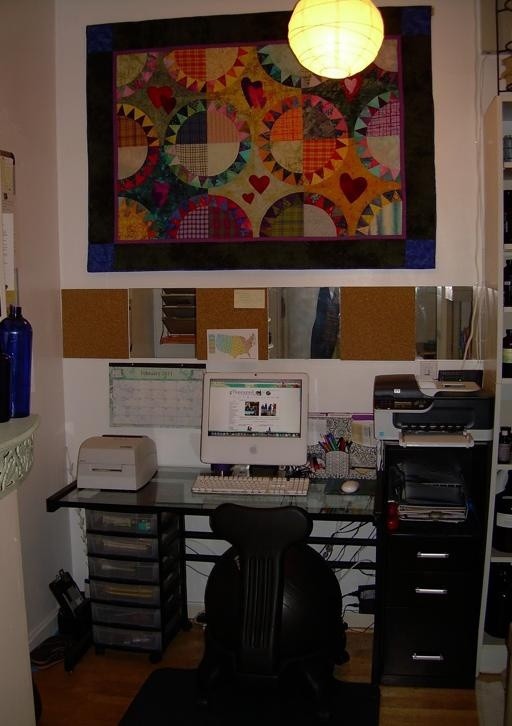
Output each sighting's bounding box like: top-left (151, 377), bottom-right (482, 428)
top-left (341, 480), bottom-right (361, 495)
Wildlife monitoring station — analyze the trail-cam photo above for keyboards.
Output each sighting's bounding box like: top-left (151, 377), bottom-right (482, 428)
top-left (191, 475), bottom-right (311, 496)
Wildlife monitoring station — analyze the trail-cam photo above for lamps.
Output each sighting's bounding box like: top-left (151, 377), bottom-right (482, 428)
top-left (283, 0), bottom-right (389, 88)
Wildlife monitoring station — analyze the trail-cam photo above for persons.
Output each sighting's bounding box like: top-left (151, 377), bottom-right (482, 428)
top-left (244, 401), bottom-right (276, 417)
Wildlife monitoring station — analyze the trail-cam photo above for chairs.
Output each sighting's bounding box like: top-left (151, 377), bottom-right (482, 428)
top-left (41, 466), bottom-right (386, 690)
top-left (191, 501), bottom-right (351, 723)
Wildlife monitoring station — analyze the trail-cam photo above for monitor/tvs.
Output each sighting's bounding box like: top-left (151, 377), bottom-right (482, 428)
top-left (199, 370), bottom-right (307, 466)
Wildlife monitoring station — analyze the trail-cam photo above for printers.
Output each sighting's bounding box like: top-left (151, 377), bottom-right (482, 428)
top-left (75, 433), bottom-right (157, 492)
top-left (372, 370), bottom-right (494, 452)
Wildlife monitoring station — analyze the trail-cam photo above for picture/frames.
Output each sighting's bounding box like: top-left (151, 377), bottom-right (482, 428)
top-left (82, 0), bottom-right (441, 271)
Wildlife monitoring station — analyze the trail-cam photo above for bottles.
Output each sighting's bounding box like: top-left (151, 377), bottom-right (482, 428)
top-left (0, 353), bottom-right (13, 423)
top-left (0, 305), bottom-right (33, 419)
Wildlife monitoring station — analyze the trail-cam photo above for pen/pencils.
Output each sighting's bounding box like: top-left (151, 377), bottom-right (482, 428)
top-left (318, 433), bottom-right (353, 453)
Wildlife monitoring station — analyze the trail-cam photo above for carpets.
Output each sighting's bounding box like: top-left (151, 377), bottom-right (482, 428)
top-left (110, 666), bottom-right (384, 726)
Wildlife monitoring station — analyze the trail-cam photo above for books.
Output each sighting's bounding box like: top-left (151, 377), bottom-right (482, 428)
top-left (398, 482), bottom-right (467, 524)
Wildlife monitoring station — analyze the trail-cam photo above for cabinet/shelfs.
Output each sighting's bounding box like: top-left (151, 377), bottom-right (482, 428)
top-left (377, 531), bottom-right (477, 697)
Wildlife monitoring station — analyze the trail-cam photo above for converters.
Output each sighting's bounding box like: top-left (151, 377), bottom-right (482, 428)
top-left (358, 584), bottom-right (377, 599)
top-left (359, 599), bottom-right (374, 614)
